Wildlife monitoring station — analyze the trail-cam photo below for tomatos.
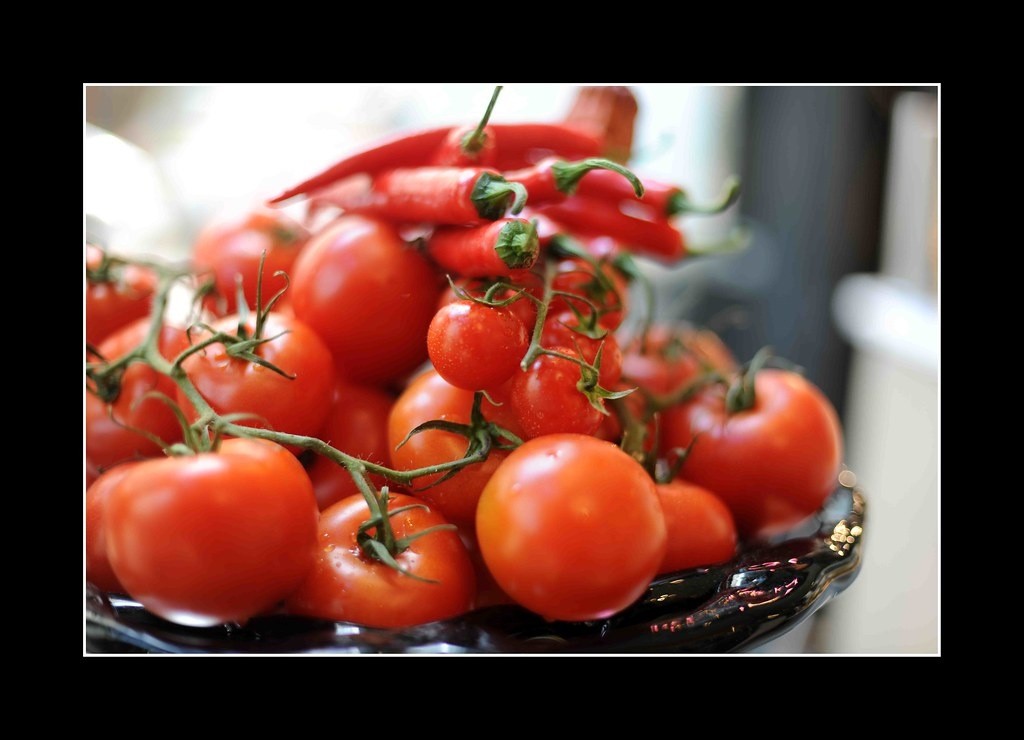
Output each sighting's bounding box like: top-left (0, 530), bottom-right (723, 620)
top-left (87, 207), bottom-right (842, 625)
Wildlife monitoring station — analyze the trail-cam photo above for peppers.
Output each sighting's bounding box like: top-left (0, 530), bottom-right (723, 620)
top-left (263, 84), bottom-right (753, 278)
